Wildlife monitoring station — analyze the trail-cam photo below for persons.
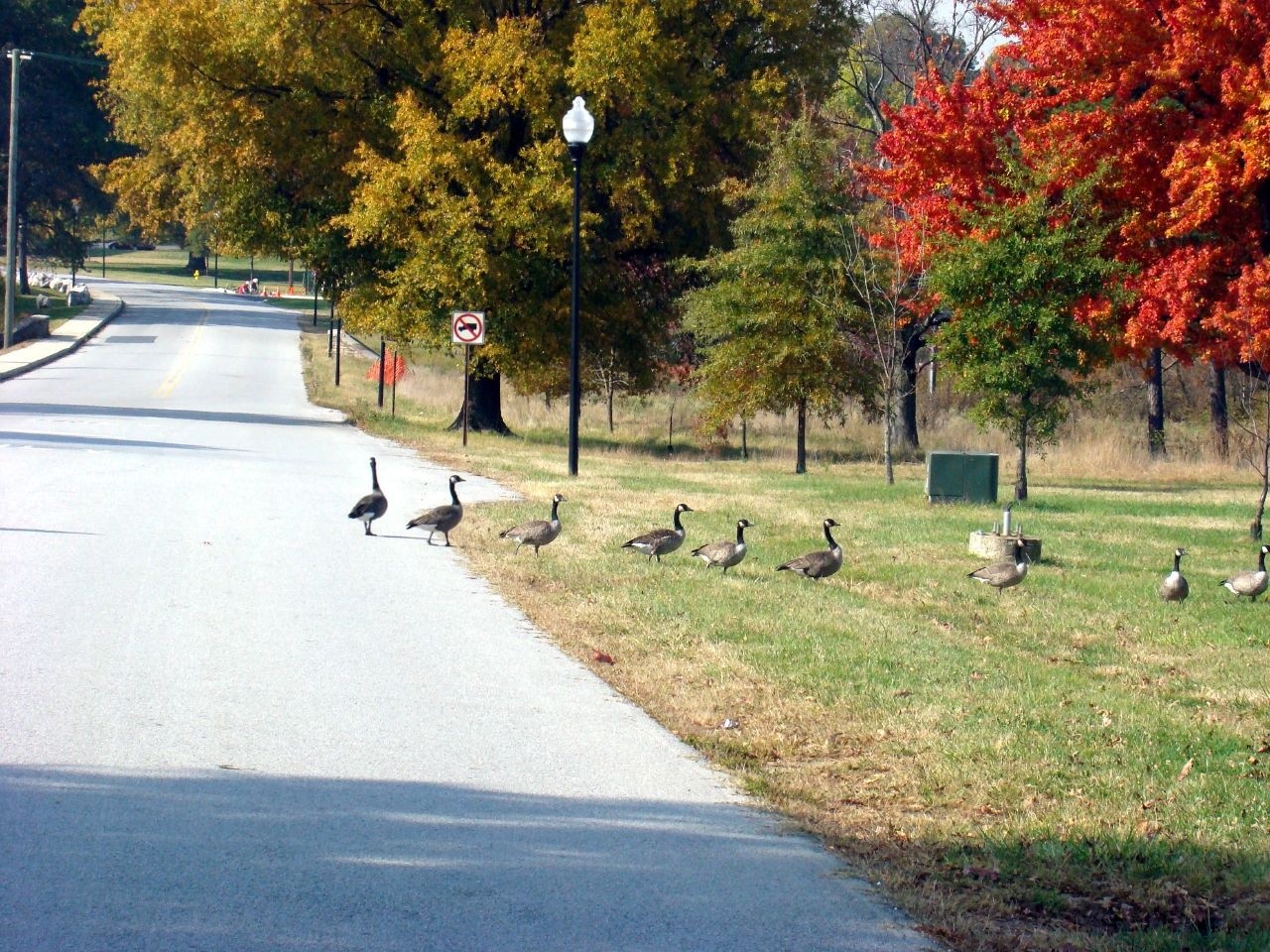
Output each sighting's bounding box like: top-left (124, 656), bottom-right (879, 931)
top-left (238, 278), bottom-right (258, 294)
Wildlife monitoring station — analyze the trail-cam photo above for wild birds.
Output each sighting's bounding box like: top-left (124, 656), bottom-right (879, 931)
top-left (623, 503), bottom-right (695, 563)
top-left (404, 474), bottom-right (467, 545)
top-left (1216, 545), bottom-right (1270, 601)
top-left (692, 519), bottom-right (755, 572)
top-left (966, 537), bottom-right (1035, 593)
top-left (1160, 547), bottom-right (1191, 603)
top-left (499, 494), bottom-right (567, 558)
top-left (348, 456), bottom-right (388, 535)
top-left (776, 519), bottom-right (843, 580)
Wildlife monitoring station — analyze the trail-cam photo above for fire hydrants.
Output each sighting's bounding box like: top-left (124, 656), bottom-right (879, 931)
top-left (194, 269), bottom-right (199, 280)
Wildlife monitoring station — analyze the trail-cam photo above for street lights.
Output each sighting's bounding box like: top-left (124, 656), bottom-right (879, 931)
top-left (562, 96), bottom-right (594, 476)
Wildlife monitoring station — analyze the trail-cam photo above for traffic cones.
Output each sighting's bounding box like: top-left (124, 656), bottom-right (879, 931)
top-left (259, 286), bottom-right (268, 297)
top-left (273, 285), bottom-right (281, 298)
top-left (289, 287), bottom-right (295, 295)
